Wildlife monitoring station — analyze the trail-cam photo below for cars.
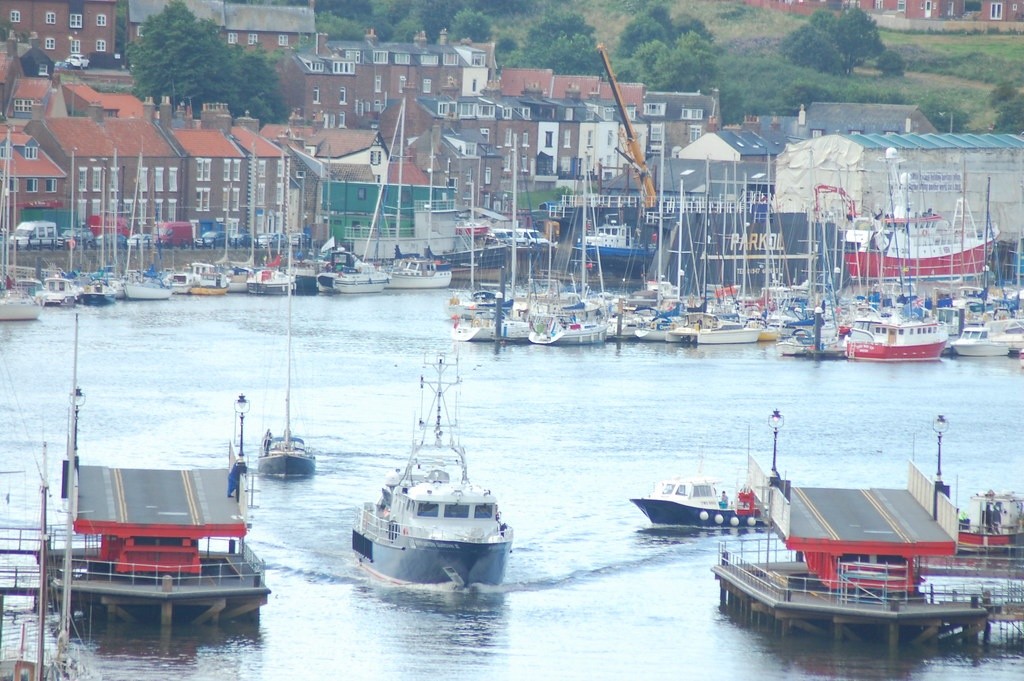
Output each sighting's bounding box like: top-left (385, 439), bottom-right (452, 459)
top-left (230, 234), bottom-right (251, 249)
top-left (195, 231), bottom-right (230, 249)
top-left (289, 233), bottom-right (306, 246)
top-left (491, 228), bottom-right (524, 246)
top-left (254, 233), bottom-right (288, 249)
top-left (96, 233), bottom-right (128, 250)
top-left (514, 227), bottom-right (549, 247)
top-left (127, 233), bottom-right (152, 248)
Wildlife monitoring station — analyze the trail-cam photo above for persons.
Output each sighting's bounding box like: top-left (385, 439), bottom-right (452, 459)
top-left (992, 505), bottom-right (1003, 535)
top-left (719, 491), bottom-right (728, 508)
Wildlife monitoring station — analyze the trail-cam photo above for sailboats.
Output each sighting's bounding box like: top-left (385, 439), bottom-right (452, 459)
top-left (0, 129), bottom-right (453, 321)
top-left (445, 134), bottom-right (1023, 354)
top-left (257, 240), bottom-right (317, 479)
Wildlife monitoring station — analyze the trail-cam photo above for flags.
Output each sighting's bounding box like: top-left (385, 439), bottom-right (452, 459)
top-left (716, 284), bottom-right (740, 297)
top-left (839, 326), bottom-right (851, 334)
top-left (266, 255), bottom-right (282, 267)
top-left (228, 444), bottom-right (243, 497)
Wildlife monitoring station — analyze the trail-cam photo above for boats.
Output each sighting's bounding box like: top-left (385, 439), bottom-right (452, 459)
top-left (668, 313), bottom-right (762, 344)
top-left (844, 320), bottom-right (949, 362)
top-left (350, 350), bottom-right (515, 591)
top-left (627, 475), bottom-right (767, 530)
top-left (951, 326), bottom-right (1011, 355)
top-left (958, 489), bottom-right (1024, 552)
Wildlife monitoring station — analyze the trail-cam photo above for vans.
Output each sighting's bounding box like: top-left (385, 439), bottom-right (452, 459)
top-left (9, 220), bottom-right (57, 250)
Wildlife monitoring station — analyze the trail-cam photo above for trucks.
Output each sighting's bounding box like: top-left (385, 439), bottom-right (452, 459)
top-left (152, 222), bottom-right (193, 249)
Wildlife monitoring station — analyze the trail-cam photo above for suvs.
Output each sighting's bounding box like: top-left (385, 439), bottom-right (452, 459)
top-left (57, 228), bottom-right (95, 250)
